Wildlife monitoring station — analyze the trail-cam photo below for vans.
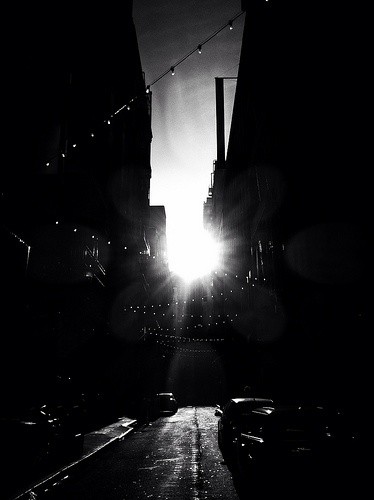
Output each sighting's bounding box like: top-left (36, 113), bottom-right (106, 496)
top-left (216, 397), bottom-right (353, 497)
top-left (154, 392), bottom-right (179, 415)
